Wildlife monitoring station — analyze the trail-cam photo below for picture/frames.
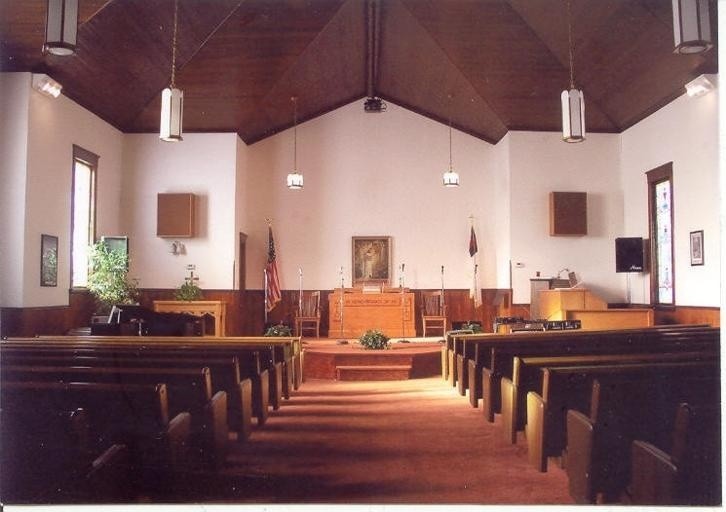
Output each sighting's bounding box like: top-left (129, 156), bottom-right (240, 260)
top-left (40, 234), bottom-right (58, 287)
top-left (690, 230), bottom-right (704, 266)
top-left (351, 236), bottom-right (392, 288)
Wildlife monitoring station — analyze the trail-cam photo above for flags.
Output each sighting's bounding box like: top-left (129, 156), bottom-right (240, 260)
top-left (265, 226), bottom-right (281, 312)
top-left (469, 229), bottom-right (478, 256)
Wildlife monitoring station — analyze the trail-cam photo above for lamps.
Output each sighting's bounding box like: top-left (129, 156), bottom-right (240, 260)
top-left (672, 0), bottom-right (714, 56)
top-left (158, 1), bottom-right (184, 143)
top-left (441, 94), bottom-right (460, 187)
top-left (560, 0), bottom-right (586, 143)
top-left (286, 96), bottom-right (304, 190)
top-left (32, 72), bottom-right (63, 99)
top-left (43, 0), bottom-right (79, 57)
top-left (683, 73), bottom-right (717, 100)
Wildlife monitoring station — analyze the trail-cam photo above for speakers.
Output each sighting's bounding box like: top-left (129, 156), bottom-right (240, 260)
top-left (102, 235), bottom-right (129, 275)
top-left (617, 236), bottom-right (644, 273)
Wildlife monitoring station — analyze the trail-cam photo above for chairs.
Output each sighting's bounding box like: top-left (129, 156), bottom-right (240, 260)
top-left (419, 290), bottom-right (448, 338)
top-left (294, 290), bottom-right (321, 340)
top-left (549, 279), bottom-right (571, 290)
top-left (360, 282), bottom-right (385, 294)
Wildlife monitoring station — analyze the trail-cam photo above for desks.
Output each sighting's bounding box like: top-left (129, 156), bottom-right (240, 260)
top-left (328, 293), bottom-right (417, 339)
top-left (152, 300), bottom-right (227, 336)
top-left (535, 289), bottom-right (608, 320)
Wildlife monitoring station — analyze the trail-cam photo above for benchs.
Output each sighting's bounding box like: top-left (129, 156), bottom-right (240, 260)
top-left (440, 323), bottom-right (720, 506)
top-left (0, 334), bottom-right (306, 504)
